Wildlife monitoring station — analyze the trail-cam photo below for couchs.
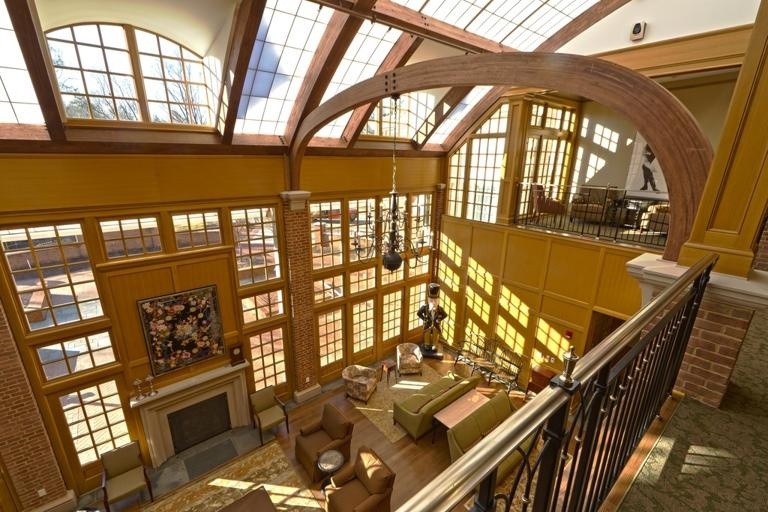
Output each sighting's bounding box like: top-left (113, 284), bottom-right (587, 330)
top-left (393, 369), bottom-right (541, 491)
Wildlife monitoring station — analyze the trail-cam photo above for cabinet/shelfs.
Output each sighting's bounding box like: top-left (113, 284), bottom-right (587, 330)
top-left (526, 366), bottom-right (557, 395)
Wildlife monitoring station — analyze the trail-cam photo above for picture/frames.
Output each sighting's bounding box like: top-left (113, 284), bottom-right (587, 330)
top-left (622, 129), bottom-right (670, 200)
top-left (135, 283), bottom-right (226, 379)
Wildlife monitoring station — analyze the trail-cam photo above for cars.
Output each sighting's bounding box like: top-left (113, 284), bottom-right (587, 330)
top-left (313, 208), bottom-right (358, 221)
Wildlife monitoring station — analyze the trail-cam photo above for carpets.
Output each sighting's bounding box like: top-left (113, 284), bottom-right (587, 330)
top-left (463, 443), bottom-right (573, 512)
top-left (120, 441), bottom-right (325, 512)
top-left (346, 358), bottom-right (442, 444)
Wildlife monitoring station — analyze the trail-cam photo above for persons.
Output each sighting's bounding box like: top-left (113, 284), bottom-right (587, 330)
top-left (638, 144), bottom-right (660, 192)
top-left (417, 282), bottom-right (447, 351)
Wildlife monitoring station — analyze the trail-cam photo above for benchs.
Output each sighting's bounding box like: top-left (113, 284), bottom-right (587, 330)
top-left (454, 340), bottom-right (520, 395)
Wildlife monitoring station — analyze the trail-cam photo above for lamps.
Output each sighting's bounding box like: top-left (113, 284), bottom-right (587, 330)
top-left (352, 116), bottom-right (435, 274)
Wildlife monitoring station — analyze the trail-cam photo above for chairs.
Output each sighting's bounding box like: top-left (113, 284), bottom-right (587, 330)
top-left (529, 181), bottom-right (670, 238)
top-left (100, 440), bottom-right (154, 512)
top-left (249, 336), bottom-right (423, 512)
